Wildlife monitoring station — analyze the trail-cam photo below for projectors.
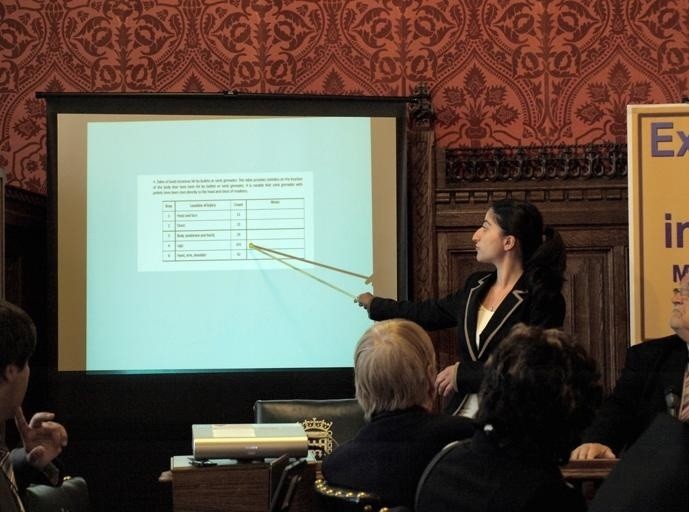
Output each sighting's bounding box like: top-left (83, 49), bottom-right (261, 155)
top-left (191, 422), bottom-right (309, 460)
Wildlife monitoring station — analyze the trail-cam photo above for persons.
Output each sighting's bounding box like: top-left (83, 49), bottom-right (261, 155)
top-left (357, 196), bottom-right (565, 421)
top-left (590, 361), bottom-right (689, 512)
top-left (566, 268), bottom-right (688, 461)
top-left (0, 302), bottom-right (70, 512)
top-left (414, 321), bottom-right (605, 511)
top-left (304, 317), bottom-right (477, 511)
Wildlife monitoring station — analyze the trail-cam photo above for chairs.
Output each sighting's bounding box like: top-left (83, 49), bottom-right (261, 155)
top-left (252, 394), bottom-right (366, 453)
